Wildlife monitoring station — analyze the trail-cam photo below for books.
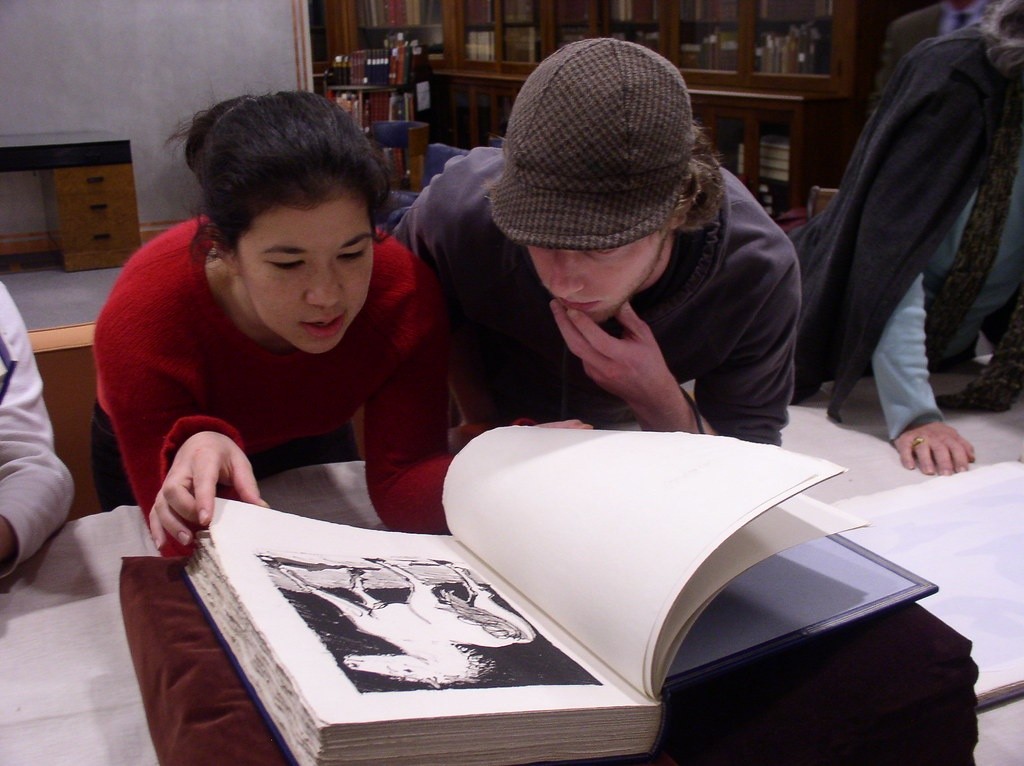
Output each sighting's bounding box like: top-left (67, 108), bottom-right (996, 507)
top-left (466, 31), bottom-right (495, 60)
top-left (611, 0), bottom-right (658, 22)
top-left (680, 30), bottom-right (738, 72)
top-left (354, 0), bottom-right (442, 29)
top-left (556, 0), bottom-right (589, 23)
top-left (679, 0), bottom-right (740, 23)
top-left (557, 27), bottom-right (659, 50)
top-left (464, 0), bottom-right (533, 26)
top-left (327, 45), bottom-right (428, 137)
top-left (758, 0), bottom-right (833, 21)
top-left (180, 427), bottom-right (939, 766)
top-left (506, 27), bottom-right (536, 63)
top-left (736, 136), bottom-right (791, 221)
top-left (753, 26), bottom-right (822, 73)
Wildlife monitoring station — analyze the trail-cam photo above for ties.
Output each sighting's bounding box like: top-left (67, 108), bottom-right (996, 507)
top-left (951, 12), bottom-right (971, 31)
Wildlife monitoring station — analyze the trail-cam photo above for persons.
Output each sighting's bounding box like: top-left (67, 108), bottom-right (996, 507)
top-left (392, 39), bottom-right (800, 446)
top-left (0, 279), bottom-right (73, 580)
top-left (785, 0), bottom-right (1023, 475)
top-left (91, 92), bottom-right (593, 557)
top-left (880, 0), bottom-right (990, 85)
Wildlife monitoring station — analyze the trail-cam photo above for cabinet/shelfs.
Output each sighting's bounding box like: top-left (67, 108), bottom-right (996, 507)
top-left (309, 0), bottom-right (858, 210)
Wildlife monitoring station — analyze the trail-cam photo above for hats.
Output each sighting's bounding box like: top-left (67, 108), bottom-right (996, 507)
top-left (489, 36), bottom-right (693, 251)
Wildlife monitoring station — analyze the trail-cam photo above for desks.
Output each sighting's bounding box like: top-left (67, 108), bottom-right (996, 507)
top-left (0, 268), bottom-right (119, 523)
top-left (0, 378), bottom-right (1024, 766)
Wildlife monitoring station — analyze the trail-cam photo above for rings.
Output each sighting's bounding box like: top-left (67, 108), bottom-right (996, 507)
top-left (912, 437), bottom-right (924, 446)
